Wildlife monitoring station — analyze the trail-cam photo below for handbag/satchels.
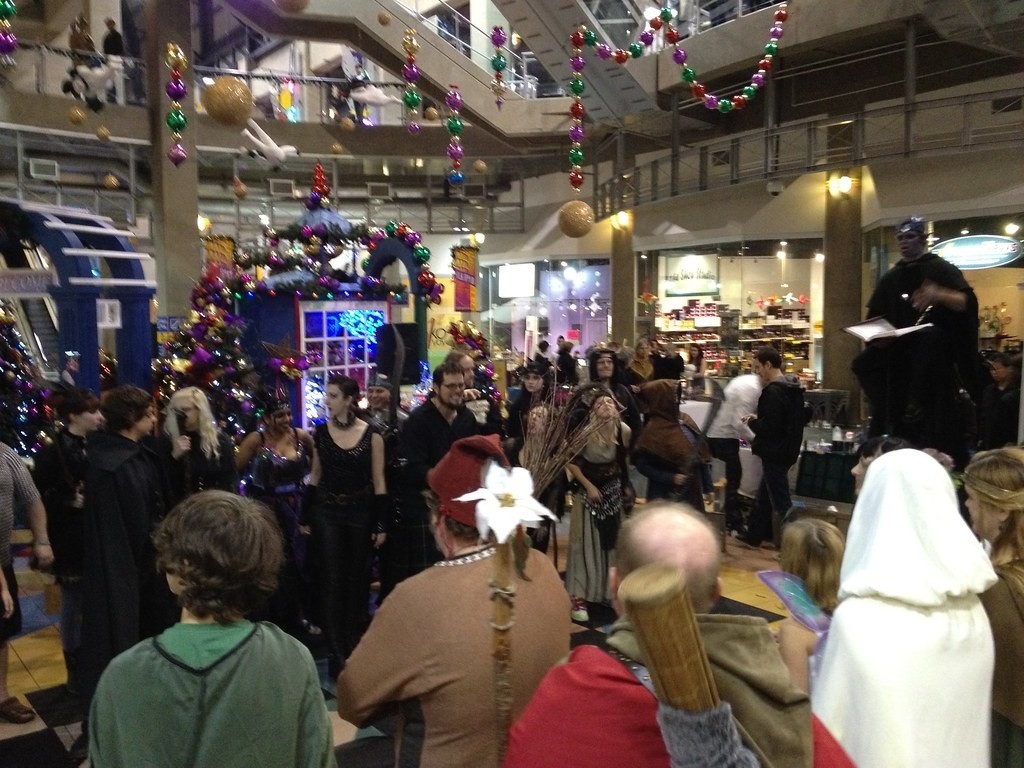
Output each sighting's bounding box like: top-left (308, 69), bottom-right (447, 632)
top-left (621, 479), bottom-right (635, 515)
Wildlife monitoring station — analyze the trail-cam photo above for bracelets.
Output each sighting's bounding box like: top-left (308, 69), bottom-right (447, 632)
top-left (32, 539), bottom-right (50, 545)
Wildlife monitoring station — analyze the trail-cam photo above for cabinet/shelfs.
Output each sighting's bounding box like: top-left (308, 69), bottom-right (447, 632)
top-left (649, 305), bottom-right (811, 376)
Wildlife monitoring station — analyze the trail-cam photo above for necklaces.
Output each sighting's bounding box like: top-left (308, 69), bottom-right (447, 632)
top-left (333, 418), bottom-right (351, 428)
top-left (453, 542), bottom-right (488, 553)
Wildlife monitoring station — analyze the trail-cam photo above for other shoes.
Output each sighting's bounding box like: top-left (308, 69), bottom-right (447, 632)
top-left (734, 534), bottom-right (760, 550)
top-left (0, 696), bottom-right (35, 723)
top-left (902, 400), bottom-right (926, 425)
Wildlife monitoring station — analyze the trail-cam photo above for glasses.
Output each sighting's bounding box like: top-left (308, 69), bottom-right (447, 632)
top-left (442, 383), bottom-right (464, 390)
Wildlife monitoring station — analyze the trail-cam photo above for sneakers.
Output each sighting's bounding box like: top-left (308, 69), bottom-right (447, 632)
top-left (566, 598), bottom-right (588, 622)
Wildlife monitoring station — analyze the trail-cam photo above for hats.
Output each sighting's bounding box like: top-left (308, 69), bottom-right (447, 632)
top-left (518, 360), bottom-right (548, 375)
top-left (428, 434), bottom-right (516, 527)
top-left (896, 218), bottom-right (925, 233)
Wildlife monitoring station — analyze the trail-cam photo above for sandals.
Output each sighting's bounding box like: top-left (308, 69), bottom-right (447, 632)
top-left (300, 619), bottom-right (323, 635)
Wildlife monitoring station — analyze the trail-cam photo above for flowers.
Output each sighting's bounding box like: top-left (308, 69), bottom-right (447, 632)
top-left (450, 459), bottom-right (560, 545)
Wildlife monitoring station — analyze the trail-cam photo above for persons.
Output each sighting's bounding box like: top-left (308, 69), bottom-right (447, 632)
top-left (44, 352), bottom-right (480, 702)
top-left (330, 65), bottom-right (369, 125)
top-left (963, 448), bottom-right (1024, 768)
top-left (852, 223), bottom-right (1023, 467)
top-left (338, 435), bottom-right (572, 768)
top-left (779, 520), bottom-right (846, 690)
top-left (815, 450), bottom-right (997, 768)
top-left (103, 19), bottom-right (123, 103)
top-left (88, 492), bottom-right (338, 768)
top-left (851, 437), bottom-right (911, 497)
top-left (0, 442), bottom-right (53, 720)
top-left (504, 505), bottom-right (814, 768)
top-left (506, 336), bottom-right (805, 626)
